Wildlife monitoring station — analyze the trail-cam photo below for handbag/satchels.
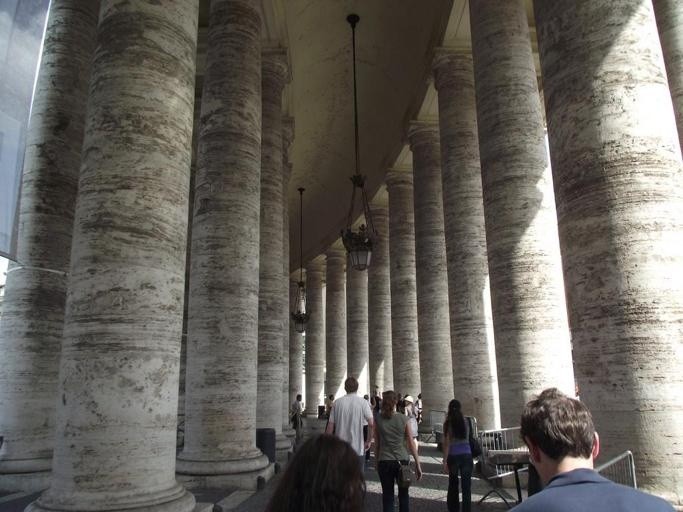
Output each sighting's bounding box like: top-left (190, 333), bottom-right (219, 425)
top-left (470, 437), bottom-right (482, 458)
top-left (398, 464), bottom-right (419, 482)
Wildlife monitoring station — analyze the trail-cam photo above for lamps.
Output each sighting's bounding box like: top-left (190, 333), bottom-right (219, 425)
top-left (341, 13), bottom-right (379, 272)
top-left (291, 187), bottom-right (311, 334)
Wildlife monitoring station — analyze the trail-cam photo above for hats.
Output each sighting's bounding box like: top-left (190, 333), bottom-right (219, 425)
top-left (404, 396), bottom-right (413, 402)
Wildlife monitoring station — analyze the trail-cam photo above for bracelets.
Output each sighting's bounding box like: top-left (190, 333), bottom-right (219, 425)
top-left (443, 460), bottom-right (447, 462)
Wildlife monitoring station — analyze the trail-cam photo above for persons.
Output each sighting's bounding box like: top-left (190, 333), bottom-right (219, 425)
top-left (397, 393), bottom-right (422, 421)
top-left (326, 395), bottom-right (334, 421)
top-left (506, 388), bottom-right (675, 512)
top-left (442, 399), bottom-right (476, 511)
top-left (374, 390), bottom-right (422, 511)
top-left (363, 391), bottom-right (382, 462)
top-left (290, 394), bottom-right (303, 448)
top-left (326, 376), bottom-right (374, 472)
top-left (266, 435), bottom-right (366, 511)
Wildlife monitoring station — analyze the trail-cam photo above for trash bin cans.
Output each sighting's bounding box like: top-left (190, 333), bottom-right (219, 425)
top-left (255, 428), bottom-right (276, 463)
top-left (318, 406), bottom-right (325, 419)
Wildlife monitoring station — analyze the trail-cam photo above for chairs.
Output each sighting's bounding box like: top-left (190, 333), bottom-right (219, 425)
top-left (425, 408), bottom-right (638, 509)
